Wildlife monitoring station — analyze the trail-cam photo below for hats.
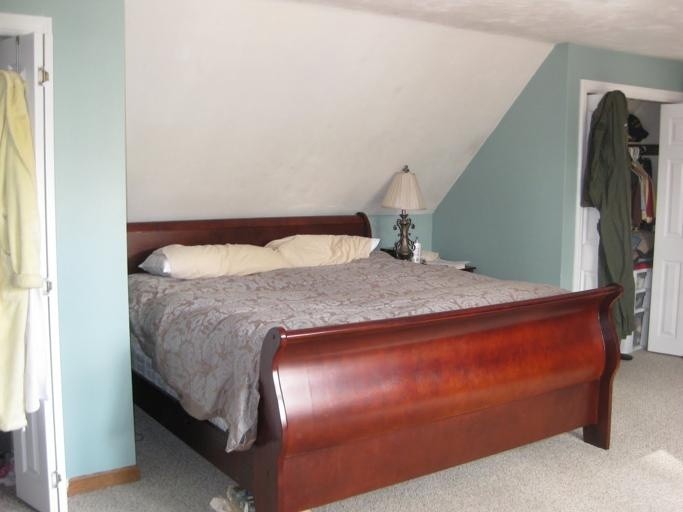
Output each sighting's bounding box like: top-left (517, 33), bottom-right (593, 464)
top-left (629, 114), bottom-right (648, 140)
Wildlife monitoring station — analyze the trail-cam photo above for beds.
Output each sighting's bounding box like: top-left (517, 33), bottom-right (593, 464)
top-left (126, 211), bottom-right (624, 512)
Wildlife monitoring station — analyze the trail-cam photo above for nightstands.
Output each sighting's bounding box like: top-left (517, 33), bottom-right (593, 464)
top-left (377, 247), bottom-right (476, 274)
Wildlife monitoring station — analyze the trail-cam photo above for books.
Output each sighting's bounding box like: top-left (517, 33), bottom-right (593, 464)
top-left (425, 261), bottom-right (469, 270)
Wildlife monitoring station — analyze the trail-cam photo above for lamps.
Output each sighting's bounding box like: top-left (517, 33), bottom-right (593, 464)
top-left (381, 164), bottom-right (428, 258)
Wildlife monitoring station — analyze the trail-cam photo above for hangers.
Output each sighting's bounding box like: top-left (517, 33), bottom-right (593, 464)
top-left (627, 147), bottom-right (651, 177)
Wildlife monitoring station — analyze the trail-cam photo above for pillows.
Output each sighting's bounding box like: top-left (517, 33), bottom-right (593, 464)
top-left (136, 242), bottom-right (288, 281)
top-left (264, 233), bottom-right (381, 269)
